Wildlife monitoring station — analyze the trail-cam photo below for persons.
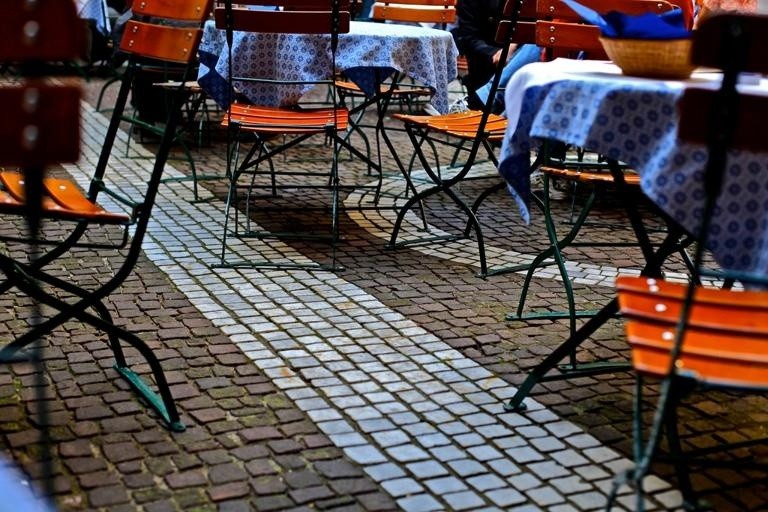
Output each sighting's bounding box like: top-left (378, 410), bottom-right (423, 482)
top-left (455, 0), bottom-right (579, 192)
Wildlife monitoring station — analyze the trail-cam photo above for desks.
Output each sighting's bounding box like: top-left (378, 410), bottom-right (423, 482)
top-left (501, 58), bottom-right (765, 414)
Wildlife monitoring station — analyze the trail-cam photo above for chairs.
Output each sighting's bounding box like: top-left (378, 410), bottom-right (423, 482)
top-left (1, 1), bottom-right (83, 511)
top-left (387, 22), bottom-right (610, 277)
top-left (509, 38), bottom-right (697, 372)
top-left (3, 1), bottom-right (214, 431)
top-left (5, 1), bottom-right (766, 195)
top-left (504, 12), bottom-right (766, 512)
top-left (210, 8), bottom-right (355, 273)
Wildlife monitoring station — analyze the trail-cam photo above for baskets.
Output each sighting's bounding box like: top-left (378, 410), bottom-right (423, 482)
top-left (597, 36), bottom-right (699, 78)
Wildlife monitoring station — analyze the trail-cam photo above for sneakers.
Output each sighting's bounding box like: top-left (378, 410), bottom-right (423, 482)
top-left (425, 96), bottom-right (471, 115)
